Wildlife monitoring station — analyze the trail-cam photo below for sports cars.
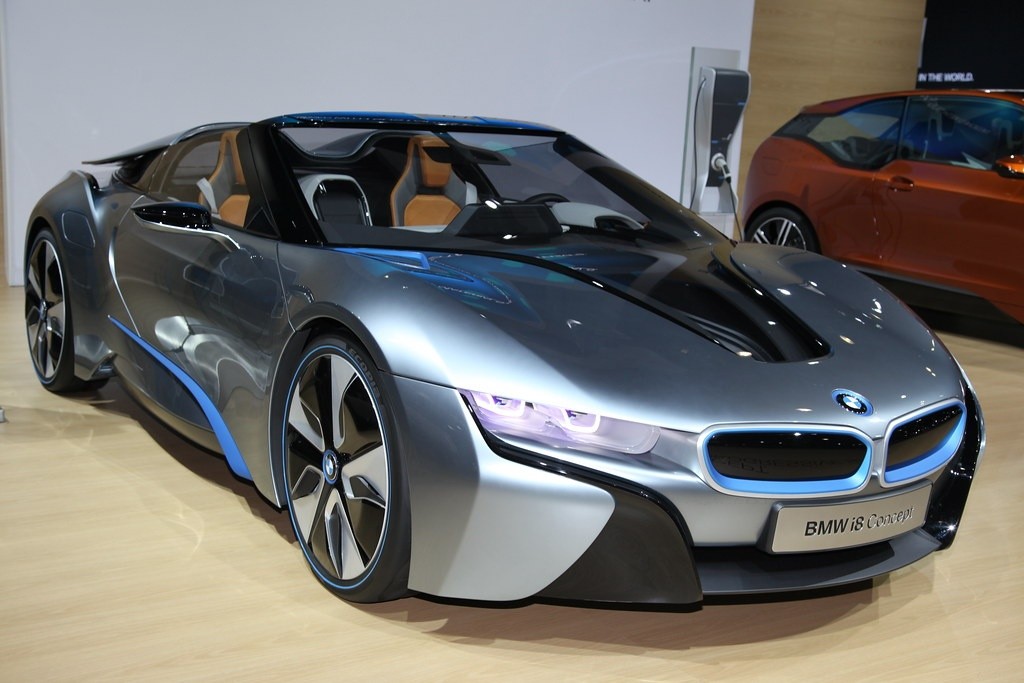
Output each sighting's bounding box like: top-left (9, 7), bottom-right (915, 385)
top-left (22, 109), bottom-right (988, 607)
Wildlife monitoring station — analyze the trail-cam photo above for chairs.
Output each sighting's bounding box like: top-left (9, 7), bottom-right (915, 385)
top-left (198, 130), bottom-right (251, 227)
top-left (390, 135), bottom-right (467, 227)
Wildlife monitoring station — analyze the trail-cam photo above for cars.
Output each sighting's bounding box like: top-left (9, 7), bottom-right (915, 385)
top-left (738, 82), bottom-right (1024, 332)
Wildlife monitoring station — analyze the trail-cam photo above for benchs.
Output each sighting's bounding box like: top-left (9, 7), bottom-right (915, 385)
top-left (824, 136), bottom-right (914, 166)
